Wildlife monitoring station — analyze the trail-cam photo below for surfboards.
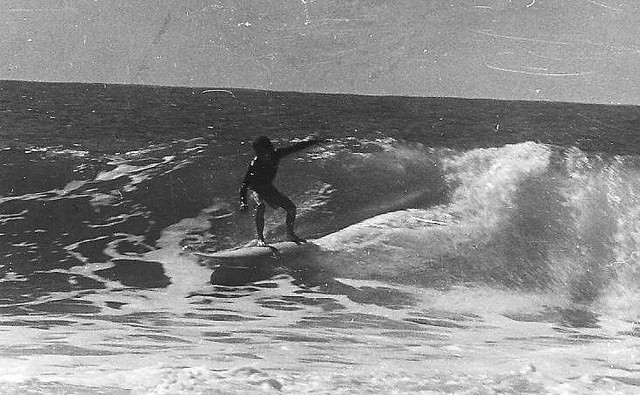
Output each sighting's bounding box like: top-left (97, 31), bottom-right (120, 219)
top-left (194, 242), bottom-right (307, 258)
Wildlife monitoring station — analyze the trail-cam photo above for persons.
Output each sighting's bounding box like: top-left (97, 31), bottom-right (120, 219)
top-left (240, 132), bottom-right (330, 247)
